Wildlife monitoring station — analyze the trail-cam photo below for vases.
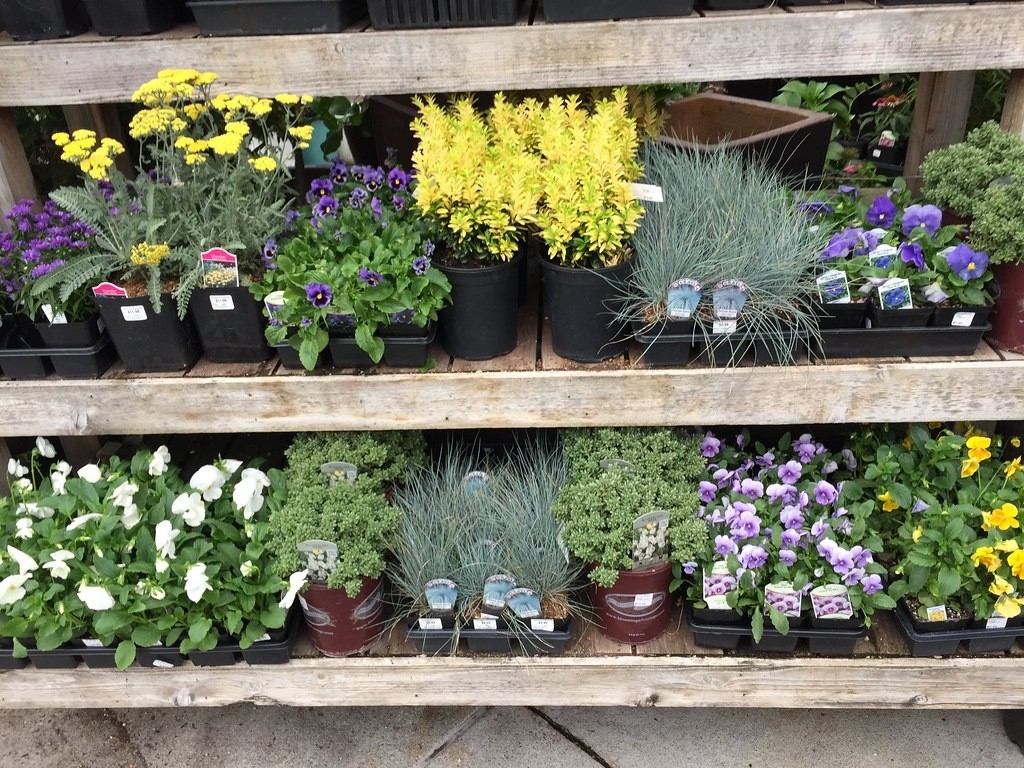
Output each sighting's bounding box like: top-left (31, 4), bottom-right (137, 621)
top-left (84, 0), bottom-right (176, 36)
top-left (24, 636), bottom-right (80, 670)
top-left (366, 0), bottom-right (522, 30)
top-left (189, 282), bottom-right (278, 363)
top-left (807, 616), bottom-right (862, 654)
top-left (749, 615), bottom-right (804, 652)
top-left (536, 244), bottom-right (636, 364)
top-left (178, 633), bottom-right (235, 667)
top-left (238, 625), bottom-right (293, 665)
top-left (860, 305), bottom-right (933, 358)
top-left (901, 597), bottom-right (970, 656)
top-left (377, 322), bottom-right (427, 368)
top-left (328, 326), bottom-right (374, 369)
top-left (542, 0), bottom-right (694, 23)
top-left (966, 611), bottom-right (1022, 652)
top-left (93, 291), bottom-right (201, 374)
top-left (135, 637), bottom-right (184, 668)
top-left (0, 320), bottom-right (55, 380)
top-left (809, 302), bottom-right (867, 359)
top-left (644, 92), bottom-right (834, 190)
top-left (31, 313), bottom-right (118, 379)
top-left (689, 601), bottom-right (746, 649)
top-left (925, 305), bottom-right (987, 356)
top-left (0, 636), bottom-right (32, 669)
top-left (431, 248), bottom-right (523, 361)
top-left (0, 0), bottom-right (89, 41)
top-left (277, 326), bottom-right (324, 370)
top-left (70, 636), bottom-right (118, 669)
top-left (184, 0), bottom-right (368, 38)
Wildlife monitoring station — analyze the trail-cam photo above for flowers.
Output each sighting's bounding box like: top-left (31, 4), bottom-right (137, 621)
top-left (0, 67), bottom-right (454, 371)
top-left (785, 182), bottom-right (994, 306)
top-left (695, 429), bottom-right (1024, 645)
top-left (531, 85), bottom-right (647, 267)
top-left (0, 438), bottom-right (310, 672)
top-left (409, 90), bottom-right (538, 265)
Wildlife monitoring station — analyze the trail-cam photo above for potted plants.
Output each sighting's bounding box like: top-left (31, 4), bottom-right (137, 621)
top-left (267, 476), bottom-right (402, 657)
top-left (549, 461), bottom-right (710, 644)
top-left (917, 120), bottom-right (1024, 355)
top-left (384, 467), bottom-right (459, 653)
top-left (575, 125), bottom-right (836, 395)
top-left (516, 490), bottom-right (606, 657)
top-left (458, 495), bottom-right (515, 652)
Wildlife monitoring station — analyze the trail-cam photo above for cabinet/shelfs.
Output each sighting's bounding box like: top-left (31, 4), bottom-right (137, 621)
top-left (0, 0), bottom-right (1024, 710)
